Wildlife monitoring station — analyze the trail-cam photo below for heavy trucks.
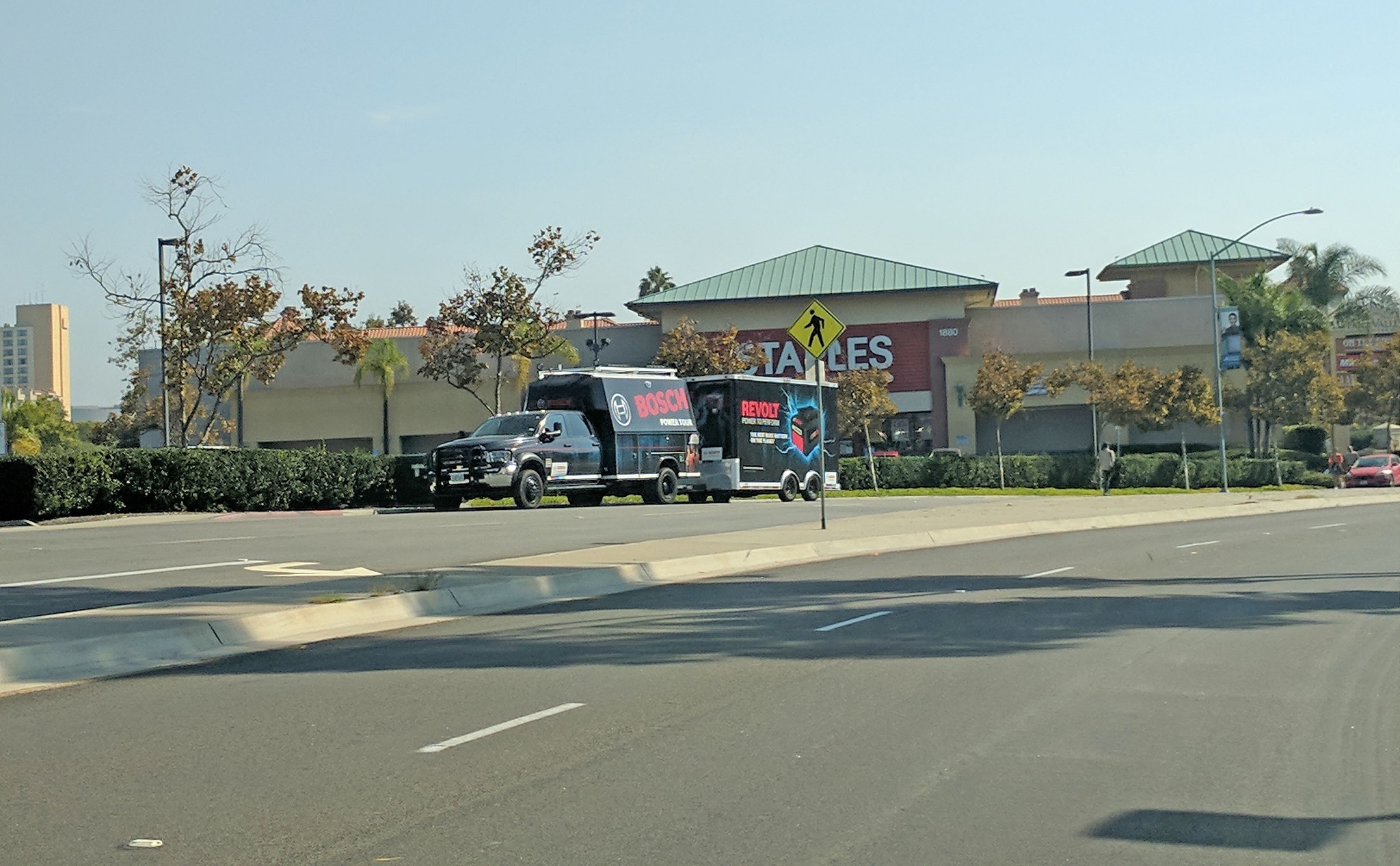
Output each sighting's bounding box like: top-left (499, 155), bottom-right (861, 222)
top-left (428, 364), bottom-right (841, 511)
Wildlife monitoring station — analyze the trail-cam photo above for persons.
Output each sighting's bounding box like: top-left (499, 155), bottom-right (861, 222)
top-left (685, 434), bottom-right (700, 472)
top-left (1344, 446), bottom-right (1358, 474)
top-left (1221, 313), bottom-right (1241, 355)
top-left (1328, 447), bottom-right (1344, 490)
top-left (1099, 442), bottom-right (1116, 496)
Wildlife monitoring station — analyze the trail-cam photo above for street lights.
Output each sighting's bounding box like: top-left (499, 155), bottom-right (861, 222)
top-left (158, 237), bottom-right (180, 448)
top-left (576, 311), bottom-right (616, 368)
top-left (1209, 208), bottom-right (1325, 494)
top-left (1064, 268), bottom-right (1099, 458)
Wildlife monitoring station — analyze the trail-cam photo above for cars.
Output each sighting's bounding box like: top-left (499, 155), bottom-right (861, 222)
top-left (1345, 454), bottom-right (1400, 488)
top-left (871, 451), bottom-right (898, 458)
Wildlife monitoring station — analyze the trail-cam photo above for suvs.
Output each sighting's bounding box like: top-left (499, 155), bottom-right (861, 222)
top-left (930, 448), bottom-right (962, 460)
